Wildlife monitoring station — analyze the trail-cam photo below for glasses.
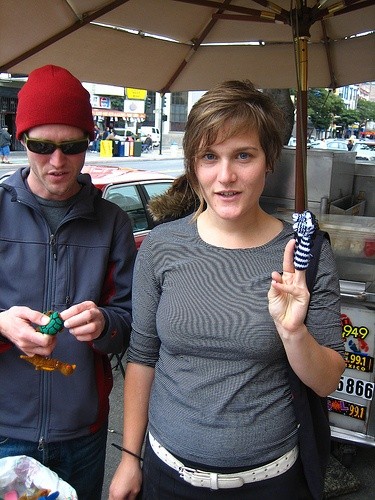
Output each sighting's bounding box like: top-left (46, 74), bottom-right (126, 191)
top-left (22, 132), bottom-right (90, 155)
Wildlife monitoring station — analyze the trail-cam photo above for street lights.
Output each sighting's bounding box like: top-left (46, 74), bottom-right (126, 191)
top-left (163, 95), bottom-right (167, 107)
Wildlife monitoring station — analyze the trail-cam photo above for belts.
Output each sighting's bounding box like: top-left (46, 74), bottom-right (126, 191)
top-left (148, 430), bottom-right (299, 489)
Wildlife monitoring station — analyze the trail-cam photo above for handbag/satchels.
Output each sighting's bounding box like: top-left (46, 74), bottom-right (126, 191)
top-left (288, 230), bottom-right (331, 500)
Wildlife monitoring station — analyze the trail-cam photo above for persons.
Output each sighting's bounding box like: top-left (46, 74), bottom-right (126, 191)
top-left (347, 140), bottom-right (353, 151)
top-left (90, 124), bottom-right (153, 153)
top-left (319, 138), bottom-right (327, 149)
top-left (306, 136), bottom-right (311, 149)
top-left (0, 125), bottom-right (13, 164)
top-left (0, 64), bottom-right (138, 500)
top-left (108, 81), bottom-right (345, 500)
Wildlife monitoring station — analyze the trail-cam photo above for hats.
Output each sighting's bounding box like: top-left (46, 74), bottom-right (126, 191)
top-left (15, 64), bottom-right (95, 142)
top-left (2, 125), bottom-right (8, 128)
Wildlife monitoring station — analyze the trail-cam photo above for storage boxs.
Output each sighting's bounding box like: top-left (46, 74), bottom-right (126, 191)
top-left (317, 214), bottom-right (375, 259)
top-left (329, 194), bottom-right (366, 216)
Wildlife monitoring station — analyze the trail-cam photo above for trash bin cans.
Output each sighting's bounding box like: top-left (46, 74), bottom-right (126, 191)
top-left (100, 139), bottom-right (142, 157)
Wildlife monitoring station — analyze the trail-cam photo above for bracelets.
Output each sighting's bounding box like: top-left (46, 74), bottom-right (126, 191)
top-left (111, 443), bottom-right (144, 461)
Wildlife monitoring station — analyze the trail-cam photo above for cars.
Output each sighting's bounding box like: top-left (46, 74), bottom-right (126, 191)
top-left (310, 140), bottom-right (349, 151)
top-left (351, 142), bottom-right (375, 160)
top-left (79, 164), bottom-right (179, 310)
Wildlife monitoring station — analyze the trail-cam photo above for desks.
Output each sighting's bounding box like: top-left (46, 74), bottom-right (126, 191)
top-left (261, 147), bottom-right (375, 217)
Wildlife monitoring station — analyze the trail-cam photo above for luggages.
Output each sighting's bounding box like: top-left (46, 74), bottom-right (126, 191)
top-left (100, 139), bottom-right (142, 158)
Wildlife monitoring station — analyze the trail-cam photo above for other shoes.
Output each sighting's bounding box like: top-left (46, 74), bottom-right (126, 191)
top-left (91, 151), bottom-right (94, 153)
top-left (5, 161), bottom-right (13, 164)
top-left (1, 161), bottom-right (6, 163)
top-left (94, 151), bottom-right (97, 153)
